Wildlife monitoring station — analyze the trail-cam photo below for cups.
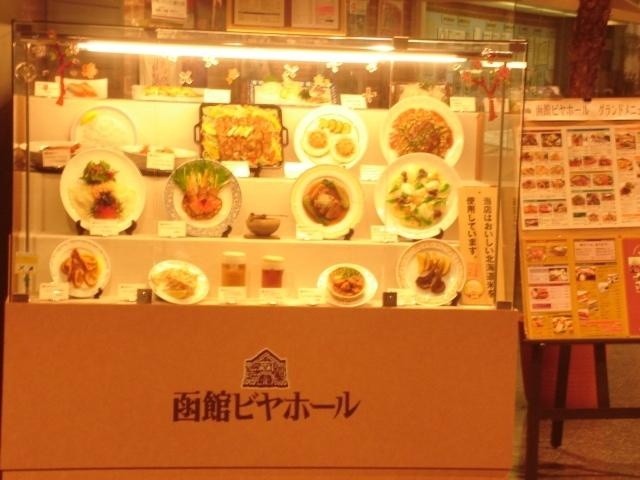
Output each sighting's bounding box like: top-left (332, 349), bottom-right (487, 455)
top-left (217, 249), bottom-right (286, 304)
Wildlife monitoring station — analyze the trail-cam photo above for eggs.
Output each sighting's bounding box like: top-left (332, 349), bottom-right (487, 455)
top-left (302, 129), bottom-right (330, 157)
top-left (330, 134), bottom-right (358, 164)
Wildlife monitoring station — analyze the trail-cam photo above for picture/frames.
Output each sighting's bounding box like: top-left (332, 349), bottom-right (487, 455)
top-left (223, 0), bottom-right (349, 39)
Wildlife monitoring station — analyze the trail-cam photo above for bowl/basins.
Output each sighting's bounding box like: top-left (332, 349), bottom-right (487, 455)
top-left (247, 218), bottom-right (281, 235)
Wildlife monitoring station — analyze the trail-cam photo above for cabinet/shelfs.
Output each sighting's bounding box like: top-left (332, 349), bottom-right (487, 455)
top-left (1, 18), bottom-right (537, 479)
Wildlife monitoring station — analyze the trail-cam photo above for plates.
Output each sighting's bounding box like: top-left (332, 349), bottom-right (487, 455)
top-left (163, 161), bottom-right (243, 237)
top-left (148, 258), bottom-right (210, 303)
top-left (292, 98), bottom-right (465, 307)
top-left (48, 103), bottom-right (149, 299)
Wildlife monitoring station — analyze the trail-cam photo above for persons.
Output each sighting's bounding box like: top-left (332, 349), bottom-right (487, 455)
top-left (248, 50), bottom-right (279, 82)
